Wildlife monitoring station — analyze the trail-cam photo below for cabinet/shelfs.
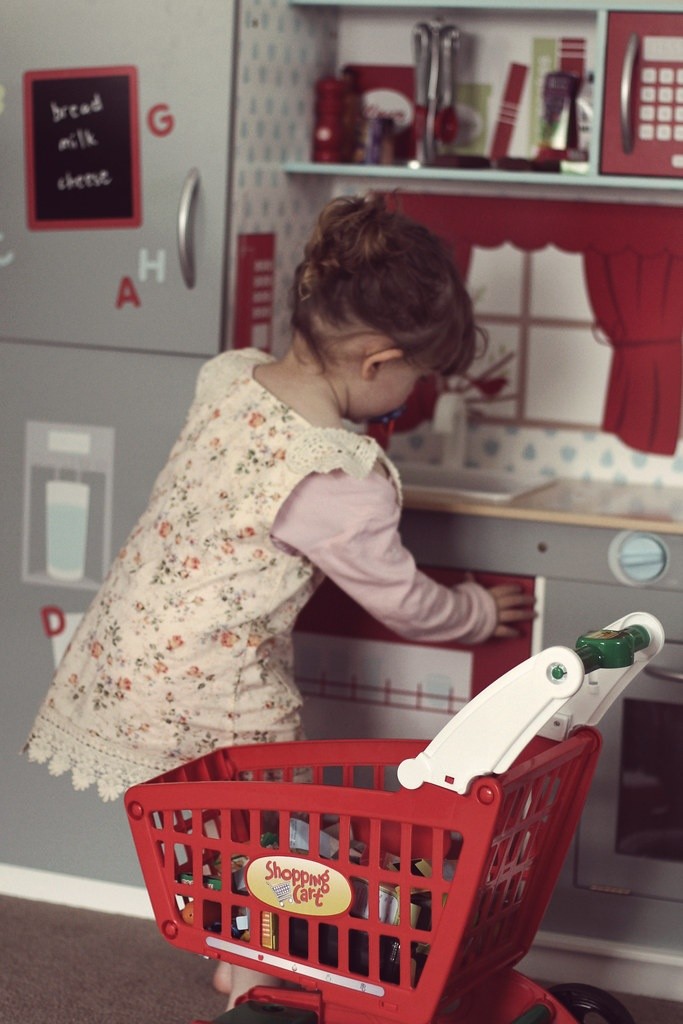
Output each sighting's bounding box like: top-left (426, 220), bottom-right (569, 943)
top-left (284, 1), bottom-right (682, 543)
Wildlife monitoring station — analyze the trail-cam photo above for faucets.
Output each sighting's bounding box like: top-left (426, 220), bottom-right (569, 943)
top-left (430, 393), bottom-right (470, 469)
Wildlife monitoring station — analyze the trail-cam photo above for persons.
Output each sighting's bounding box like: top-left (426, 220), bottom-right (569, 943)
top-left (10, 185), bottom-right (546, 1024)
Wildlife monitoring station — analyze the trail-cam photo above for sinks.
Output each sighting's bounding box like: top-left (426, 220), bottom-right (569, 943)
top-left (386, 456), bottom-right (557, 503)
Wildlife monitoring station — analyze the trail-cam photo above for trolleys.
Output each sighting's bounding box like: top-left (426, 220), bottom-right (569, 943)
top-left (122, 610), bottom-right (663, 1024)
